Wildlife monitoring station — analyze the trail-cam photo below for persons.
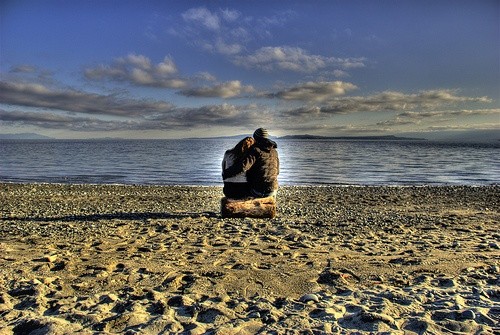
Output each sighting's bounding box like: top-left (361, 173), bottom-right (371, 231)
top-left (222, 137), bottom-right (255, 200)
top-left (222, 128), bottom-right (279, 197)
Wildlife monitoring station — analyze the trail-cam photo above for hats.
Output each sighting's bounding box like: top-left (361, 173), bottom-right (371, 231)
top-left (254, 128), bottom-right (268, 138)
